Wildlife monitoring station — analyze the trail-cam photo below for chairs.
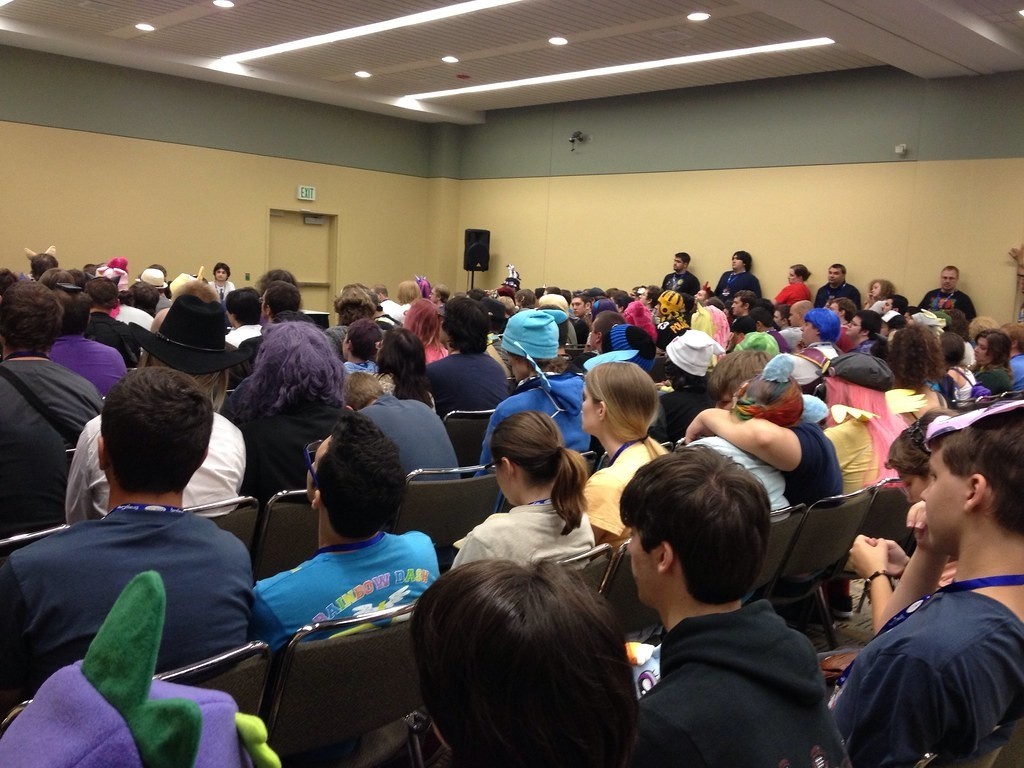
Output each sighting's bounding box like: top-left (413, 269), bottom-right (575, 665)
top-left (183, 495), bottom-right (259, 551)
top-left (913, 723), bottom-right (1002, 768)
top-left (150, 639), bottom-right (268, 716)
top-left (268, 602), bottom-right (433, 768)
top-left (799, 476), bottom-right (915, 633)
top-left (443, 408), bottom-right (494, 478)
top-left (597, 441), bottom-right (674, 470)
top-left (392, 464), bottom-right (501, 568)
top-left (675, 437), bottom-right (687, 448)
top-left (1002, 390), bottom-right (1024, 400)
top-left (566, 343), bottom-right (586, 357)
top-left (975, 394), bottom-right (1001, 404)
top-left (495, 450), bottom-right (599, 513)
top-left (763, 485), bottom-right (876, 652)
top-left (0, 524), bottom-right (71, 567)
top-left (596, 538), bottom-right (660, 643)
top-left (555, 542), bottom-right (613, 593)
top-left (252, 490), bottom-right (319, 586)
top-left (753, 501), bottom-right (806, 599)
top-left (951, 398), bottom-right (977, 410)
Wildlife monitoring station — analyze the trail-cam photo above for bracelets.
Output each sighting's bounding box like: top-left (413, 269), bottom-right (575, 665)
top-left (863, 570), bottom-right (895, 600)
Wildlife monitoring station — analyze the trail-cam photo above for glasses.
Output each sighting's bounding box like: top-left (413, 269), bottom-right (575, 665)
top-left (482, 460), bottom-right (520, 474)
top-left (303, 440), bottom-right (323, 498)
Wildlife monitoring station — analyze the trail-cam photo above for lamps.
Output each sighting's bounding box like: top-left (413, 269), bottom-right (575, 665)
top-left (568, 130), bottom-right (584, 153)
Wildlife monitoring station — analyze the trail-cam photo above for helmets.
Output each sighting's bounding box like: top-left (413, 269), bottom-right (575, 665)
top-left (804, 308), bottom-right (840, 342)
top-left (657, 290), bottom-right (683, 315)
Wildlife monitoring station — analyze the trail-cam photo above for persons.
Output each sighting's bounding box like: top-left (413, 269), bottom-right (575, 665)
top-left (0, 246), bottom-right (1024, 768)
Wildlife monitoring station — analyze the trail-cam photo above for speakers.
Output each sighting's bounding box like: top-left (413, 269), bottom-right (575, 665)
top-left (464, 229), bottom-right (490, 271)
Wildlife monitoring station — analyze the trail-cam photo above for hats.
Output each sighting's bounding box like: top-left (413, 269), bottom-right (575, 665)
top-left (501, 309), bottom-right (569, 359)
top-left (591, 299), bottom-right (618, 322)
top-left (539, 293), bottom-right (570, 314)
top-left (815, 353), bottom-right (895, 393)
top-left (135, 269), bottom-right (168, 289)
top-left (602, 324), bottom-right (655, 372)
top-left (665, 330), bottom-right (726, 377)
top-left (129, 295), bottom-right (253, 374)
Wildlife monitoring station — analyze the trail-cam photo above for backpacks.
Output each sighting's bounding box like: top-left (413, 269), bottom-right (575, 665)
top-left (951, 366), bottom-right (990, 403)
